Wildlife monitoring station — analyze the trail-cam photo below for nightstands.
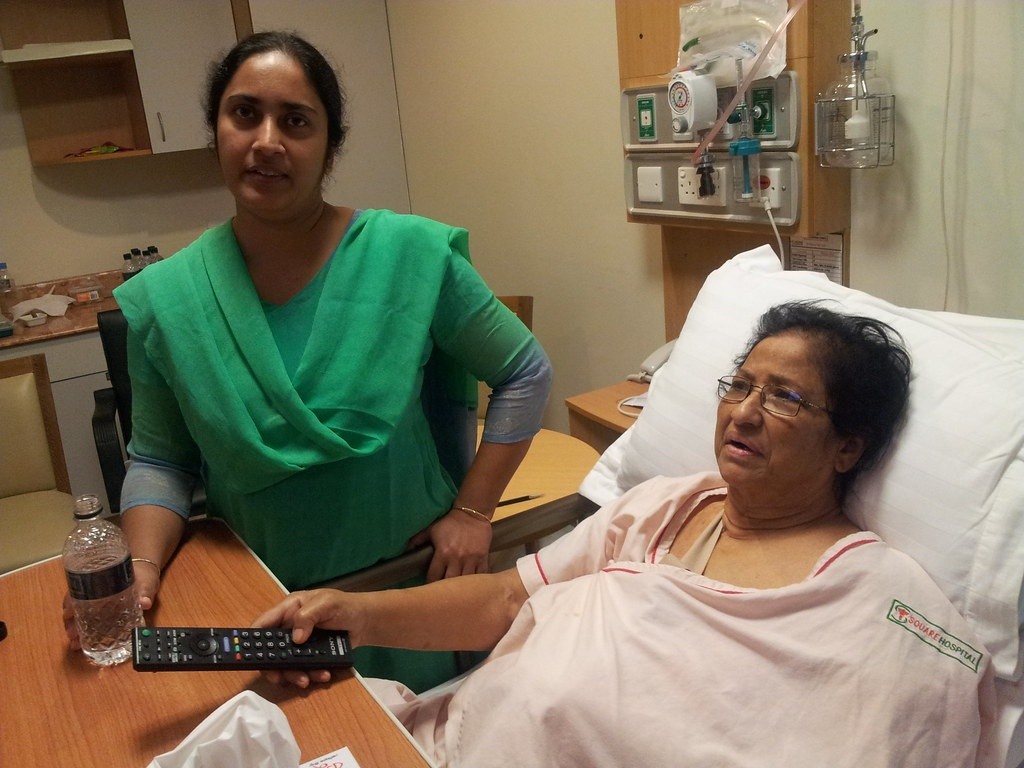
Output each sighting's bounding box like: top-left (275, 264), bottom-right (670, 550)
top-left (565, 379), bottom-right (651, 456)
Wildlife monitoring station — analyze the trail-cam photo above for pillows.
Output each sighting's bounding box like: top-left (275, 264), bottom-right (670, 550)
top-left (579, 247), bottom-right (1024, 676)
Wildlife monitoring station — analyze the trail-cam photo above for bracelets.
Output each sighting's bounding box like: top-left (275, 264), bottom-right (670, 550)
top-left (452, 505), bottom-right (491, 522)
top-left (131, 559), bottom-right (160, 576)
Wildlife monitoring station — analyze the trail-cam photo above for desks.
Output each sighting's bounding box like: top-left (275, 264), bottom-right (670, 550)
top-left (0, 516), bottom-right (440, 768)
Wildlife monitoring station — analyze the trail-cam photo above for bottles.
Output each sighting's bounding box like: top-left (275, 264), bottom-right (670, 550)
top-left (121, 246), bottom-right (164, 281)
top-left (822, 51), bottom-right (892, 166)
top-left (62, 495), bottom-right (146, 666)
top-left (1, 263), bottom-right (14, 315)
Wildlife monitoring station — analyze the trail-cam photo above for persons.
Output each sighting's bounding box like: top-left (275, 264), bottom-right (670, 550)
top-left (255, 303), bottom-right (997, 768)
top-left (63, 32), bottom-right (554, 696)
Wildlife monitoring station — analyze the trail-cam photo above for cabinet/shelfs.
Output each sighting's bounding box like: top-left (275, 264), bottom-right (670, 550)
top-left (0, 0), bottom-right (259, 165)
top-left (1, 331), bottom-right (128, 516)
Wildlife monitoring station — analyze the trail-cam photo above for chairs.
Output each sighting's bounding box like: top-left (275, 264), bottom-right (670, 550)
top-left (0, 352), bottom-right (77, 577)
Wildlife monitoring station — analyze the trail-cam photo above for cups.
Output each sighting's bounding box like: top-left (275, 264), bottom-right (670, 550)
top-left (729, 140), bottom-right (761, 203)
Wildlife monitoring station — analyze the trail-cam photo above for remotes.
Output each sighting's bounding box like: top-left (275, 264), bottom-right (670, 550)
top-left (132, 625), bottom-right (353, 672)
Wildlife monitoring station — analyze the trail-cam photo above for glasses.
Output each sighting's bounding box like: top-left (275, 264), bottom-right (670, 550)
top-left (717, 376), bottom-right (828, 431)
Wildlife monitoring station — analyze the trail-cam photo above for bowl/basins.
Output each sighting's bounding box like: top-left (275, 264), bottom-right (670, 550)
top-left (18, 312), bottom-right (48, 327)
top-left (68, 286), bottom-right (106, 306)
